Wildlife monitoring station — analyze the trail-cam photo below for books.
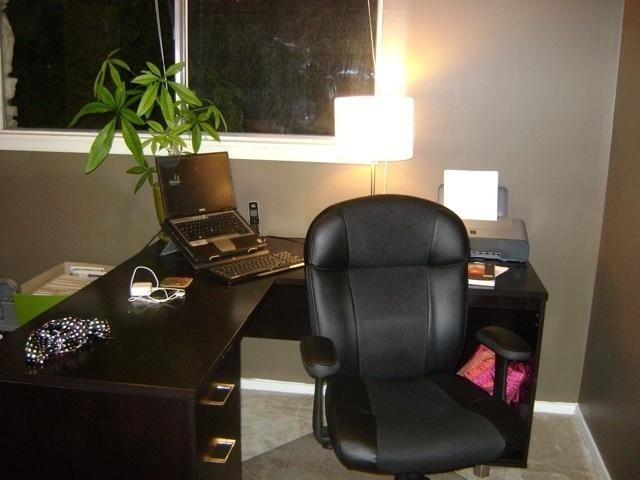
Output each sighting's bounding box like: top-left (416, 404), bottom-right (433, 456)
top-left (465, 258), bottom-right (496, 287)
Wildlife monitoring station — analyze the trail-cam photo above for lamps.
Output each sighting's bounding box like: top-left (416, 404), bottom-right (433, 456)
top-left (331, 95), bottom-right (414, 199)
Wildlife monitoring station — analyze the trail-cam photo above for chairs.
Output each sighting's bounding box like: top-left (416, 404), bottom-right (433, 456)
top-left (299, 194), bottom-right (535, 480)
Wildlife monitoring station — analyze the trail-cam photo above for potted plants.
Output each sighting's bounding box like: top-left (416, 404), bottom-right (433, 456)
top-left (68, 47), bottom-right (229, 244)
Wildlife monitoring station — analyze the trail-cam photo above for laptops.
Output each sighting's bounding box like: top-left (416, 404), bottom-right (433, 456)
top-left (156, 151), bottom-right (270, 271)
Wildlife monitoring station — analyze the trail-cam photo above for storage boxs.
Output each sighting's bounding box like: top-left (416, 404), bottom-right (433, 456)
top-left (11, 262), bottom-right (119, 326)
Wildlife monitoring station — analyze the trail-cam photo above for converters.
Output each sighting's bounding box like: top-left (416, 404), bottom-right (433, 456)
top-left (130, 282), bottom-right (151, 296)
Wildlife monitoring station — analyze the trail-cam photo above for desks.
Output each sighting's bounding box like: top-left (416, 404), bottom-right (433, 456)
top-left (0, 234), bottom-right (549, 480)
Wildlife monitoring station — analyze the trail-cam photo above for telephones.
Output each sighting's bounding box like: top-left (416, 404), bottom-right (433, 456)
top-left (248, 200), bottom-right (260, 234)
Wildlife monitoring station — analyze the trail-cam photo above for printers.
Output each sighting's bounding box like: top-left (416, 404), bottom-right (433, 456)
top-left (437, 184), bottom-right (530, 265)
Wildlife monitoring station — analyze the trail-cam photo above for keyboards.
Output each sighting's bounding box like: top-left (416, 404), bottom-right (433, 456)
top-left (208, 250), bottom-right (306, 284)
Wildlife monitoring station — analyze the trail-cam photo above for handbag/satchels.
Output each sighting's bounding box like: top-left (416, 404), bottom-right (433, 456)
top-left (459, 343), bottom-right (528, 406)
